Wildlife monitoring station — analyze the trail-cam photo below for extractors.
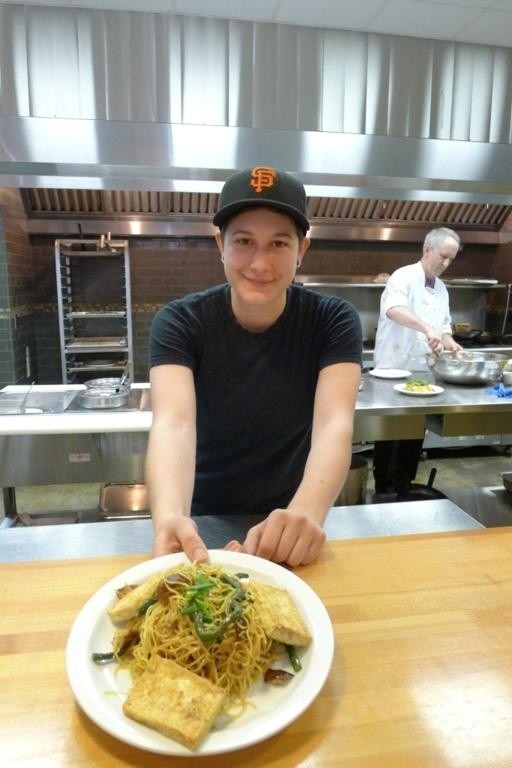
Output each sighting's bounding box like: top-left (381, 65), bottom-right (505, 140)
top-left (1, 2), bottom-right (511, 248)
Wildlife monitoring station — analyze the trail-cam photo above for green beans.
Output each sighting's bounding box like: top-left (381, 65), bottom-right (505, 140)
top-left (195, 573), bottom-right (244, 640)
top-left (287, 644), bottom-right (301, 672)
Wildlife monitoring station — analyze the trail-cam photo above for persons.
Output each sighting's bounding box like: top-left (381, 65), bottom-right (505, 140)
top-left (144, 165), bottom-right (364, 565)
top-left (372, 228), bottom-right (464, 500)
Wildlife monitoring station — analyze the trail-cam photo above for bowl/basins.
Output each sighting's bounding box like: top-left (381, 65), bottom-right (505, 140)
top-left (499, 471), bottom-right (512, 495)
top-left (423, 349), bottom-right (511, 388)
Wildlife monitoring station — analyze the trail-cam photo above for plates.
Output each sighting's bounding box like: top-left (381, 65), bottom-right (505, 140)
top-left (62, 544), bottom-right (338, 759)
top-left (392, 382), bottom-right (445, 397)
top-left (367, 367), bottom-right (413, 380)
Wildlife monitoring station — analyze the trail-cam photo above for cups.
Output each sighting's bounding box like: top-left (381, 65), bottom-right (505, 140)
top-left (502, 371), bottom-right (512, 389)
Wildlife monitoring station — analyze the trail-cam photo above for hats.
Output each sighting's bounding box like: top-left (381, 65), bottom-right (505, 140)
top-left (212, 167), bottom-right (310, 231)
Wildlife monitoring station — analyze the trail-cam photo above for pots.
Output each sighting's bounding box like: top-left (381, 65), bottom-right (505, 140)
top-left (400, 466), bottom-right (448, 502)
top-left (333, 454), bottom-right (376, 506)
top-left (76, 374), bottom-right (131, 410)
top-left (452, 329), bottom-right (512, 345)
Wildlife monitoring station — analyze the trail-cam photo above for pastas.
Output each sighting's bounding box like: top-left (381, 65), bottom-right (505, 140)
top-left (109, 558), bottom-right (283, 733)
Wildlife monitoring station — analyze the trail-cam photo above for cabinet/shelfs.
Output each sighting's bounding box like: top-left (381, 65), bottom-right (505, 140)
top-left (55, 235), bottom-right (135, 383)
